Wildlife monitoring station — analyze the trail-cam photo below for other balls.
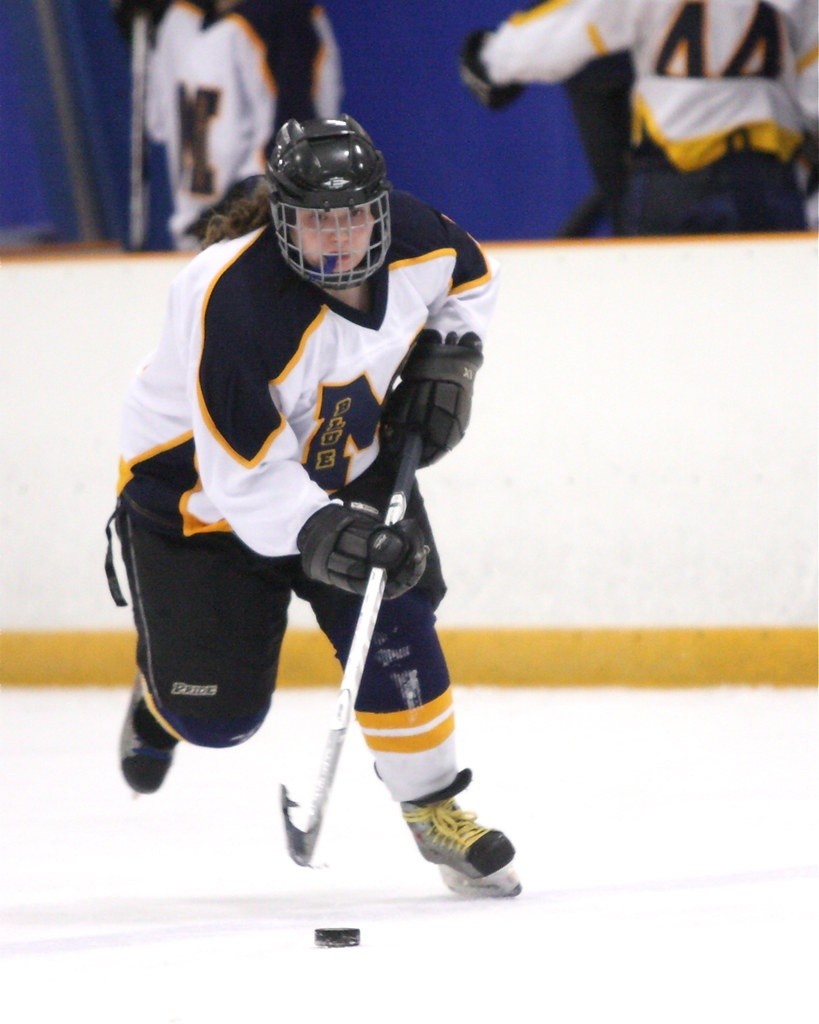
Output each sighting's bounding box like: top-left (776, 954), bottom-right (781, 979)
top-left (314, 928), bottom-right (361, 948)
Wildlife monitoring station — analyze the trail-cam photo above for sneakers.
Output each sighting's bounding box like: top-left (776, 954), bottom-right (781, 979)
top-left (400, 768), bottom-right (524, 901)
top-left (120, 673), bottom-right (180, 796)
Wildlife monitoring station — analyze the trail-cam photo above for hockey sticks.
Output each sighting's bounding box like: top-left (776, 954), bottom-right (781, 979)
top-left (278, 433), bottom-right (421, 868)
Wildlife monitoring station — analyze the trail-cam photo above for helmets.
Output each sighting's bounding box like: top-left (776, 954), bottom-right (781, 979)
top-left (264, 112), bottom-right (392, 291)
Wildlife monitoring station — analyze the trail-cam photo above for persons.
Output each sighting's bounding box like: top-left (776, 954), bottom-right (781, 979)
top-left (125, 0), bottom-right (340, 249)
top-left (460, 0), bottom-right (818, 237)
top-left (104, 117), bottom-right (520, 903)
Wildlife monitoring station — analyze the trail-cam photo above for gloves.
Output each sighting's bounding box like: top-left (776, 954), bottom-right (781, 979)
top-left (297, 503), bottom-right (429, 601)
top-left (457, 27), bottom-right (528, 110)
top-left (377, 327), bottom-right (484, 475)
